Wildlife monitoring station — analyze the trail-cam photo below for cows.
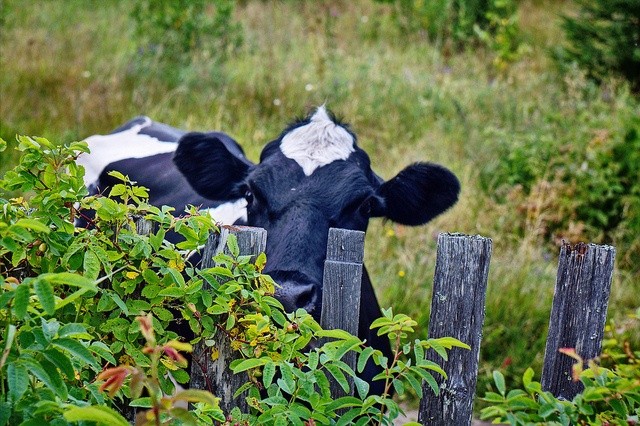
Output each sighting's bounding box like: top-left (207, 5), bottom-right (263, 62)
top-left (66, 102), bottom-right (463, 426)
